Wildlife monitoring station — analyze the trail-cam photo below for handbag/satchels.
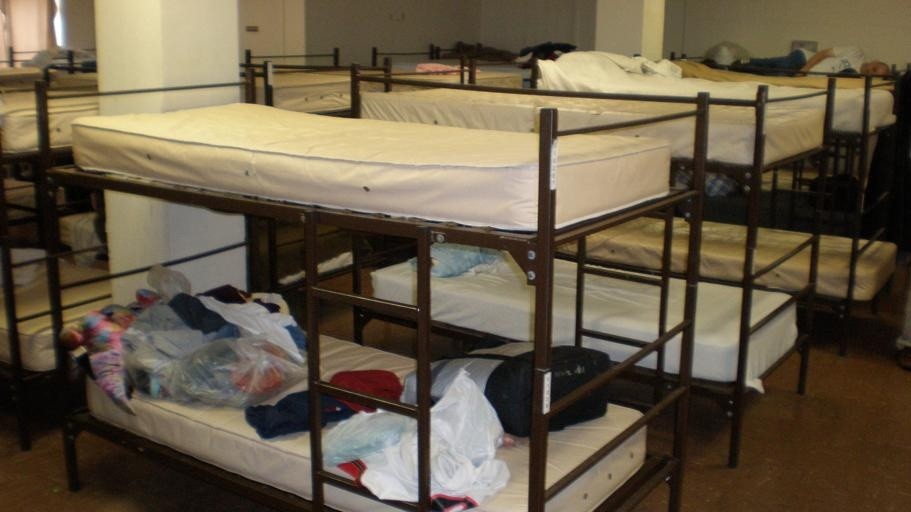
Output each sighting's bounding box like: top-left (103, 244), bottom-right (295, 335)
top-left (397, 340), bottom-right (608, 438)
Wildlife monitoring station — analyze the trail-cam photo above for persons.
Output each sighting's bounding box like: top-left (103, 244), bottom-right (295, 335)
top-left (715, 43), bottom-right (892, 79)
